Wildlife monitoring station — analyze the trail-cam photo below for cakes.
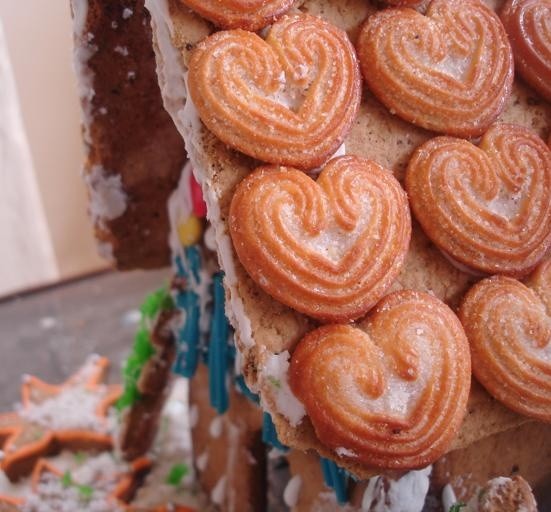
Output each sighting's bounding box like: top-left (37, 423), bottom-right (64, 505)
top-left (0, 1), bottom-right (551, 512)
